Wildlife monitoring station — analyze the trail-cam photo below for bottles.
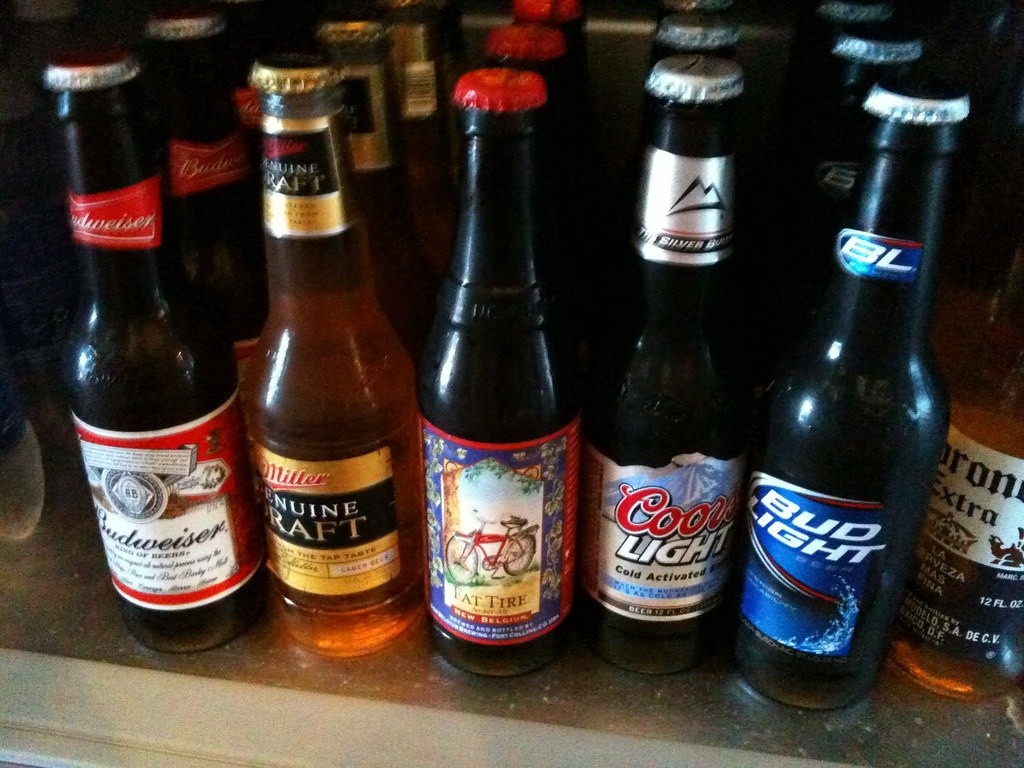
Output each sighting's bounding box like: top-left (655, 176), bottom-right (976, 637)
top-left (2, 1), bottom-right (1024, 716)
